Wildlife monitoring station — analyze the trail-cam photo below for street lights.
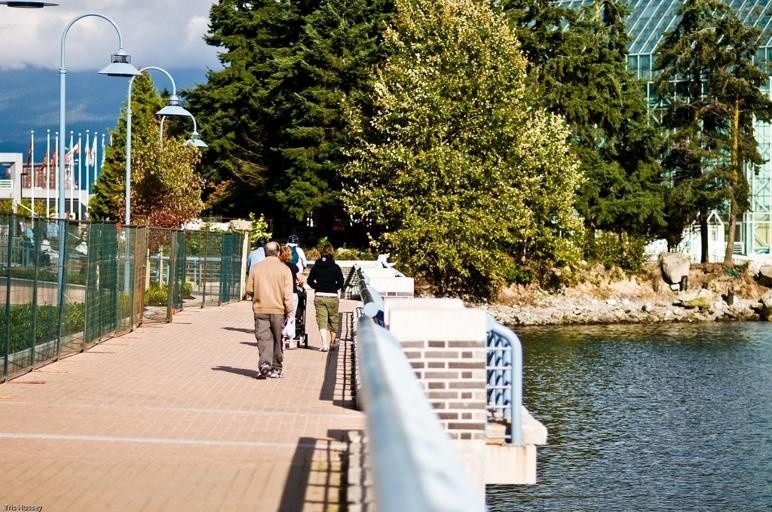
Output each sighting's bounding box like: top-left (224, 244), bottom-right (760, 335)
top-left (146, 109), bottom-right (211, 292)
top-left (52, 11), bottom-right (143, 316)
top-left (122, 62), bottom-right (192, 299)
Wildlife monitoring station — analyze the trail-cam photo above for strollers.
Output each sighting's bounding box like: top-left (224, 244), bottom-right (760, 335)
top-left (293, 283), bottom-right (310, 348)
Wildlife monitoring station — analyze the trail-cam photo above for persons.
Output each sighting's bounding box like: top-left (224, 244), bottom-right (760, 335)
top-left (307, 243), bottom-right (345, 353)
top-left (244, 240), bottom-right (295, 380)
top-left (275, 246), bottom-right (304, 351)
top-left (246, 235), bottom-right (267, 278)
top-left (284, 232), bottom-right (307, 280)
top-left (74, 230), bottom-right (90, 278)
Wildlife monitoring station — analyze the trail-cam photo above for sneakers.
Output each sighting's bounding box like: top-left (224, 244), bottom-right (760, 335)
top-left (256, 362), bottom-right (273, 379)
top-left (269, 368), bottom-right (284, 378)
top-left (320, 341), bottom-right (337, 353)
top-left (284, 338), bottom-right (298, 350)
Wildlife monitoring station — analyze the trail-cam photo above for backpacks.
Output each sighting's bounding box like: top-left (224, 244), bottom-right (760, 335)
top-left (288, 245), bottom-right (299, 264)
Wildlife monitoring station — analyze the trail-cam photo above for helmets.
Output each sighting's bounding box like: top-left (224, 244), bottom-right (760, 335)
top-left (288, 234), bottom-right (299, 244)
top-left (256, 237), bottom-right (268, 246)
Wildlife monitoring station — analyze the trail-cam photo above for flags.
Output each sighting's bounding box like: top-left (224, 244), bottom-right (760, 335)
top-left (37, 150), bottom-right (48, 173)
top-left (22, 136), bottom-right (35, 182)
top-left (51, 132), bottom-right (109, 168)
